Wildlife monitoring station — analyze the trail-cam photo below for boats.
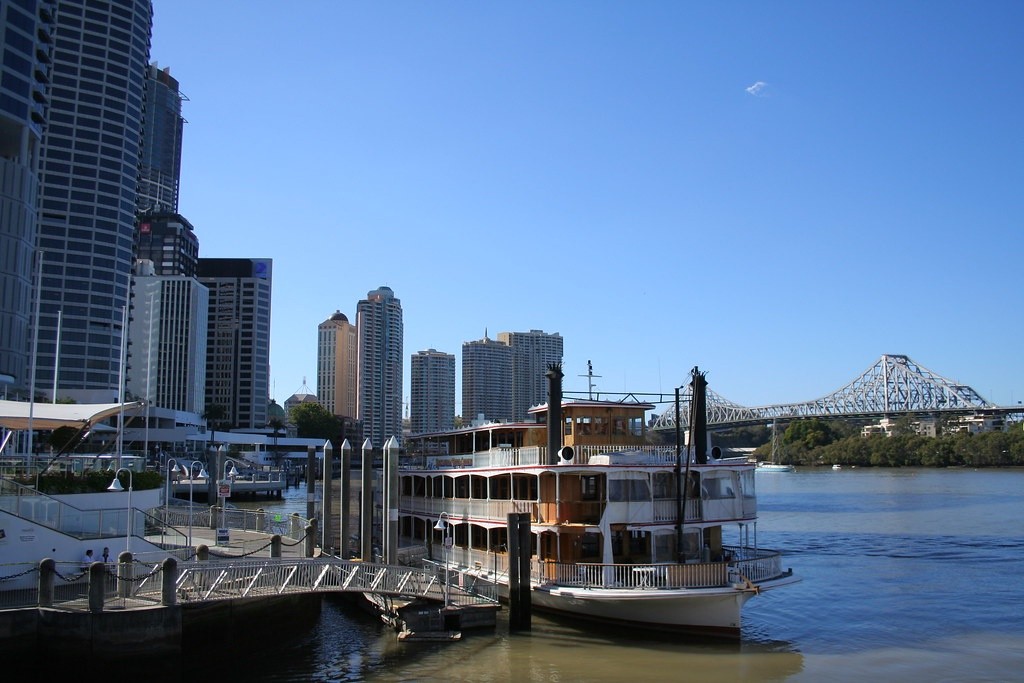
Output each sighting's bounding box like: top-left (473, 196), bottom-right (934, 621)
top-left (744, 459), bottom-right (795, 472)
top-left (372, 361), bottom-right (802, 638)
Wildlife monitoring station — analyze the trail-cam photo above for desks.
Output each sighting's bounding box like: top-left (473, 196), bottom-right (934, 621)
top-left (633, 567), bottom-right (656, 588)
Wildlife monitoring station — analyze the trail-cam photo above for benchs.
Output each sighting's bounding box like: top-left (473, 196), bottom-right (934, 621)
top-left (67, 568), bottom-right (118, 576)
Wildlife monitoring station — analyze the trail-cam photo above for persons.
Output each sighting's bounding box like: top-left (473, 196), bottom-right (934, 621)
top-left (81, 548), bottom-right (94, 589)
top-left (99, 546), bottom-right (116, 586)
